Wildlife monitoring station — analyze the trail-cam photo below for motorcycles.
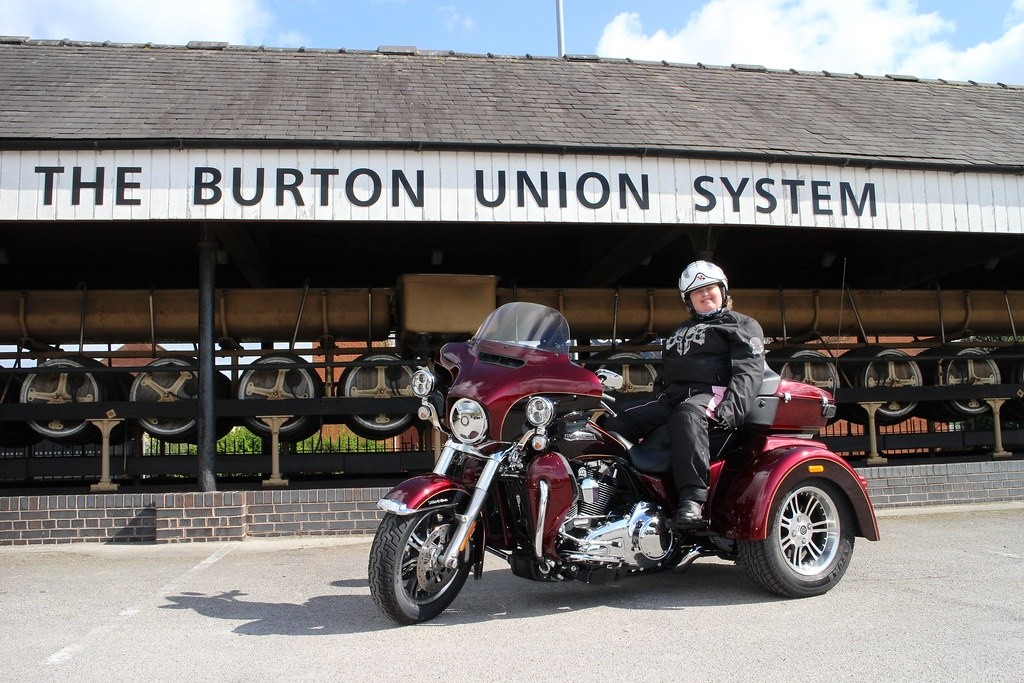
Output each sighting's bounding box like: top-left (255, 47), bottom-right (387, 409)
top-left (367, 301), bottom-right (881, 627)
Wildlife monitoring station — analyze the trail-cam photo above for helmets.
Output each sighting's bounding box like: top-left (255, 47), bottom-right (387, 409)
top-left (678, 260), bottom-right (729, 303)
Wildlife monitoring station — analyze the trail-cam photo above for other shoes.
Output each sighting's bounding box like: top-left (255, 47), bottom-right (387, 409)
top-left (676, 499), bottom-right (705, 524)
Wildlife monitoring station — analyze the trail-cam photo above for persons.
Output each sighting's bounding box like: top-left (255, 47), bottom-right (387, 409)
top-left (604, 260), bottom-right (765, 524)
